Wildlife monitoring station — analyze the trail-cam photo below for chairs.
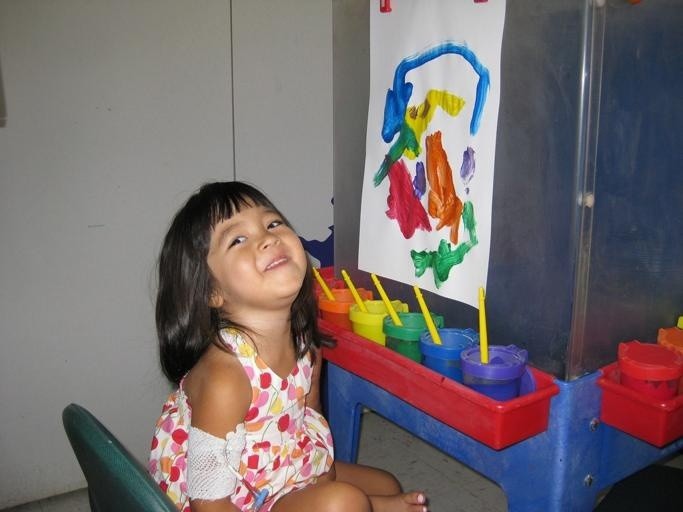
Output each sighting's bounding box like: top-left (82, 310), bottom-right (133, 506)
top-left (63, 403), bottom-right (180, 512)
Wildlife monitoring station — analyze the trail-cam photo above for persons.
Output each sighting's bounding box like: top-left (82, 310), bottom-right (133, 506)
top-left (147, 180), bottom-right (429, 512)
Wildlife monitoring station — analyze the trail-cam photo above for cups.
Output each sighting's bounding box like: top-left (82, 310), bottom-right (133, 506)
top-left (615, 315), bottom-right (683, 403)
top-left (307, 260), bottom-right (530, 404)
